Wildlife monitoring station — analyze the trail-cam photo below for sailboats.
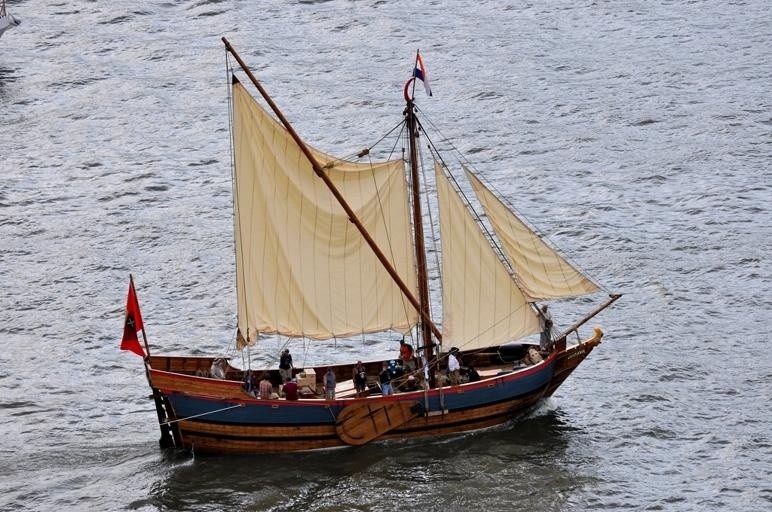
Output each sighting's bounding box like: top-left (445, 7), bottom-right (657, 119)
top-left (120, 29), bottom-right (624, 458)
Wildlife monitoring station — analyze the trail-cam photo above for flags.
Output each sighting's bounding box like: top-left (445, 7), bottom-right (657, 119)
top-left (120, 279), bottom-right (146, 357)
top-left (412, 55), bottom-right (434, 97)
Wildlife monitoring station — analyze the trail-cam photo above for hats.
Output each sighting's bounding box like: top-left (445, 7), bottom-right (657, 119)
top-left (212, 357), bottom-right (221, 365)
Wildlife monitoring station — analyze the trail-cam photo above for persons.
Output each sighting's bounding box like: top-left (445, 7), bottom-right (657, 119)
top-left (279, 349), bottom-right (293, 382)
top-left (323, 366), bottom-right (336, 400)
top-left (447, 346), bottom-right (464, 385)
top-left (259, 373), bottom-right (273, 399)
top-left (282, 376), bottom-right (298, 400)
top-left (211, 358), bottom-right (226, 380)
top-left (379, 361), bottom-right (394, 396)
top-left (536, 303), bottom-right (553, 351)
top-left (352, 361), bottom-right (366, 398)
top-left (398, 340), bottom-right (416, 371)
top-left (242, 369), bottom-right (257, 398)
